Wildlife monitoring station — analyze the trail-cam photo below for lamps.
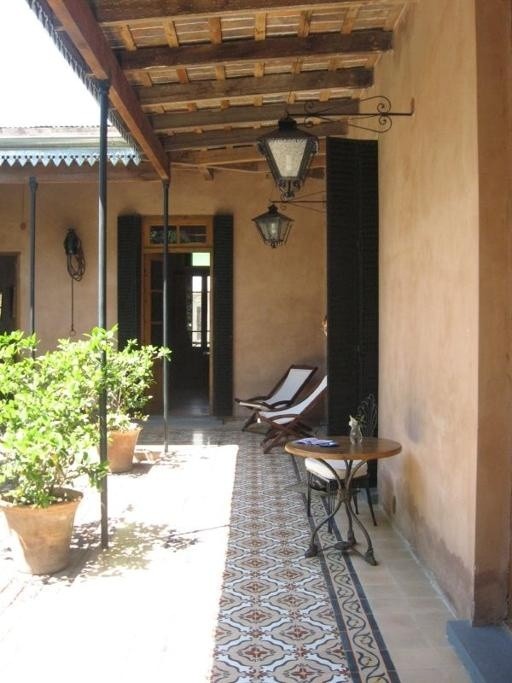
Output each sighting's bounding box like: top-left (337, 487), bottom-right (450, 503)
top-left (250, 192), bottom-right (380, 249)
top-left (258, 93), bottom-right (414, 198)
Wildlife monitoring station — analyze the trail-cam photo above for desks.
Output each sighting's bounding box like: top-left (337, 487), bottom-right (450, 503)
top-left (285, 432), bottom-right (402, 568)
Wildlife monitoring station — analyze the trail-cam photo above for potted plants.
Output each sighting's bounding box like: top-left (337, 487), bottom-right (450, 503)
top-left (0, 321), bottom-right (112, 577)
top-left (87, 335), bottom-right (169, 473)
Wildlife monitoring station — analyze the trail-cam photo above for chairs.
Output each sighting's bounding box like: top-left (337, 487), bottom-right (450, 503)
top-left (256, 378), bottom-right (327, 454)
top-left (303, 393), bottom-right (380, 533)
top-left (234, 362), bottom-right (317, 436)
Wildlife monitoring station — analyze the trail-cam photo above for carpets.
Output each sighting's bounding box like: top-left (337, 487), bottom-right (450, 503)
top-left (0, 425), bottom-right (406, 683)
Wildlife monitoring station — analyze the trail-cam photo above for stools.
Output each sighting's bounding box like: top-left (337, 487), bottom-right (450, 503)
top-left (306, 457), bottom-right (377, 532)
